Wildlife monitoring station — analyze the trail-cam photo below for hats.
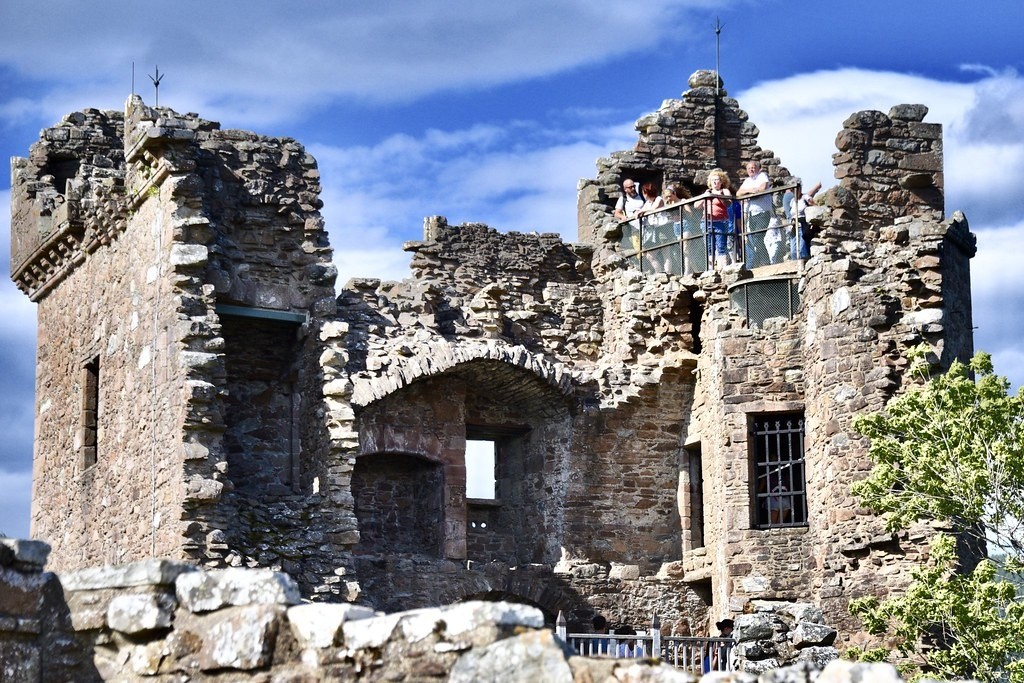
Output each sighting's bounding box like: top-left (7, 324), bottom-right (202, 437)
top-left (716, 619), bottom-right (734, 630)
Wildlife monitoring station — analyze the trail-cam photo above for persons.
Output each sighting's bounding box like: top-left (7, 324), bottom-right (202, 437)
top-left (758, 467), bottom-right (791, 523)
top-left (613, 179), bottom-right (821, 275)
top-left (585, 616), bottom-right (735, 674)
top-left (736, 161), bottom-right (773, 269)
top-left (694, 171), bottom-right (731, 269)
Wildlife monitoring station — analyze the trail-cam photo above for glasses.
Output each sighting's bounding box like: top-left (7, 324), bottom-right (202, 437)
top-left (626, 185), bottom-right (636, 189)
